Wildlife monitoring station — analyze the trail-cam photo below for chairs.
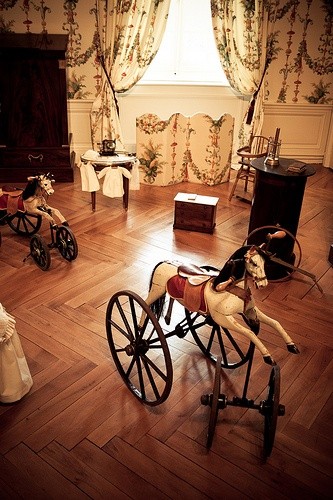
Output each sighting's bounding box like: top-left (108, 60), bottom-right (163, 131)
top-left (227, 132), bottom-right (275, 205)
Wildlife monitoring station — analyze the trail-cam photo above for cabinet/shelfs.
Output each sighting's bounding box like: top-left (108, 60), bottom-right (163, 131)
top-left (0, 32), bottom-right (75, 183)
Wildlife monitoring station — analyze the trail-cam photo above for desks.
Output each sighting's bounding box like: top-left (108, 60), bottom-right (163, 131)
top-left (246, 159), bottom-right (317, 281)
top-left (80, 150), bottom-right (137, 213)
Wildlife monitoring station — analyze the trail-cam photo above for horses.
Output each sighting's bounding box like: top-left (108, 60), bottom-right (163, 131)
top-left (0, 171), bottom-right (70, 230)
top-left (136, 242), bottom-right (300, 366)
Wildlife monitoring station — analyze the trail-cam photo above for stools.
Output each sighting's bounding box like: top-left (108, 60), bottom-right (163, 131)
top-left (173, 191), bottom-right (220, 235)
